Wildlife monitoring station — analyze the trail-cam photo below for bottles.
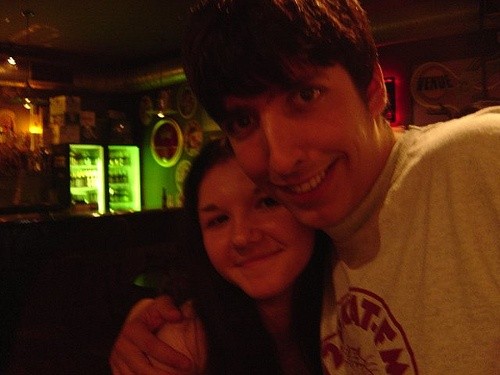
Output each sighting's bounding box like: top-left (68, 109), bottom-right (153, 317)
top-left (70, 148), bottom-right (132, 203)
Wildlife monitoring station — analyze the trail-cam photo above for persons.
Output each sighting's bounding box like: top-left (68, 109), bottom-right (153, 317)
top-left (143, 136), bottom-right (338, 374)
top-left (110, 1), bottom-right (499, 374)
